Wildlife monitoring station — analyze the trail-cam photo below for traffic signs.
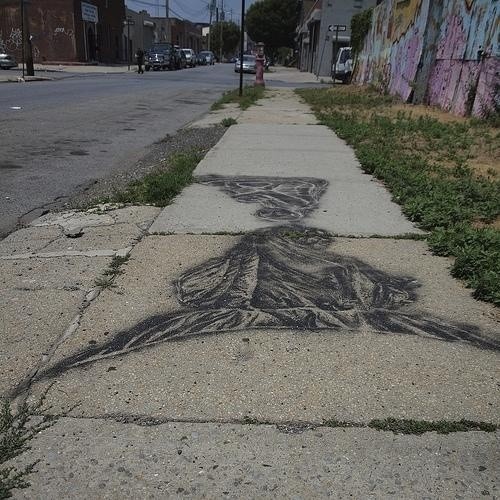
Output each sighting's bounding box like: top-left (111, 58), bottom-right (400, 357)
top-left (122, 20), bottom-right (134, 26)
top-left (329, 25), bottom-right (346, 31)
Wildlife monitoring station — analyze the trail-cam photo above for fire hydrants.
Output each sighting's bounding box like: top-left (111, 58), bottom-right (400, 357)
top-left (255, 42), bottom-right (266, 87)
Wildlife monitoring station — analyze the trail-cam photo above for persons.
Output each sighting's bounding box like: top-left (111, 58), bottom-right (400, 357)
top-left (135, 48), bottom-right (144, 74)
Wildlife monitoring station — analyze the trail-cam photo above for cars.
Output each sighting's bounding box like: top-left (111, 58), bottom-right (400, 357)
top-left (0, 48), bottom-right (17, 70)
top-left (175, 45), bottom-right (215, 68)
top-left (234, 54), bottom-right (270, 72)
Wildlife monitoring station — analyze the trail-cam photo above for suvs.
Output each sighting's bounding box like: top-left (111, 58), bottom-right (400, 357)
top-left (331, 46), bottom-right (353, 80)
top-left (145, 43), bottom-right (175, 70)
